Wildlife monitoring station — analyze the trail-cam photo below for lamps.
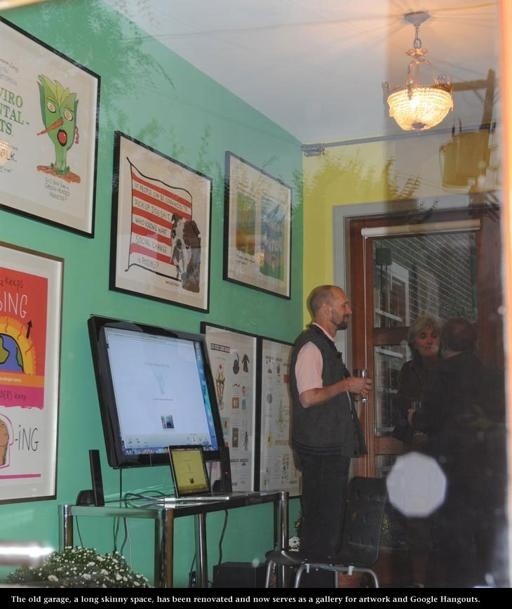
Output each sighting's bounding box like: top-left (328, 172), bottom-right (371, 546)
top-left (388, 12), bottom-right (454, 133)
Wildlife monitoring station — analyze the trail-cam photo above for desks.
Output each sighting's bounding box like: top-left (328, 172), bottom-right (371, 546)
top-left (56, 479), bottom-right (288, 590)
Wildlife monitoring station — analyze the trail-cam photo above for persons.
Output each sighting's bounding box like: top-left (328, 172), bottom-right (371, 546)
top-left (286, 285), bottom-right (374, 589)
top-left (384, 316), bottom-right (444, 589)
top-left (404, 317), bottom-right (494, 589)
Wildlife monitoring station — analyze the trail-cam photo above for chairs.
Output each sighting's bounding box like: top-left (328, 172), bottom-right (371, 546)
top-left (261, 474), bottom-right (389, 589)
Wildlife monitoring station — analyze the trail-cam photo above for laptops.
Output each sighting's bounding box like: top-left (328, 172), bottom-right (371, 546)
top-left (167, 445), bottom-right (261, 500)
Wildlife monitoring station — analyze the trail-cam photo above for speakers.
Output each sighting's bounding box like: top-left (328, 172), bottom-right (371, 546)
top-left (76, 449), bottom-right (105, 507)
top-left (212, 447), bottom-right (232, 493)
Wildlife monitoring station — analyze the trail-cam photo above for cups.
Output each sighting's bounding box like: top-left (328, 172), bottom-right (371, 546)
top-left (354, 368), bottom-right (368, 401)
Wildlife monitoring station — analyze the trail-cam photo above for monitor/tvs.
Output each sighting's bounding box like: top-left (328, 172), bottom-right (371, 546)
top-left (88, 316), bottom-right (229, 468)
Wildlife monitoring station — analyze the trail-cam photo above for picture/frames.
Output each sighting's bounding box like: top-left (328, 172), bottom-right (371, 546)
top-left (199, 320), bottom-right (257, 494)
top-left (111, 131), bottom-right (213, 313)
top-left (0, 240), bottom-right (64, 504)
top-left (258, 335), bottom-right (305, 499)
top-left (222, 151), bottom-right (292, 298)
top-left (0, 16), bottom-right (102, 236)
